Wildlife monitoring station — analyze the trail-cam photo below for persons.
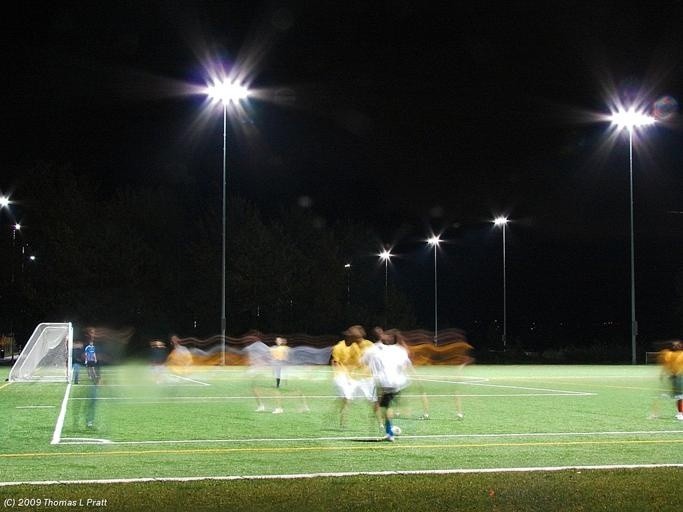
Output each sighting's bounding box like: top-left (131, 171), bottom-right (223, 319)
top-left (656, 339), bottom-right (682, 422)
top-left (69, 322), bottom-right (472, 441)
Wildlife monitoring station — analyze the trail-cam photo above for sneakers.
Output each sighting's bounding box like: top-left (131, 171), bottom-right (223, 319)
top-left (254, 404), bottom-right (312, 415)
top-left (394, 412), bottom-right (464, 420)
top-left (673, 412), bottom-right (683, 421)
top-left (376, 434), bottom-right (394, 442)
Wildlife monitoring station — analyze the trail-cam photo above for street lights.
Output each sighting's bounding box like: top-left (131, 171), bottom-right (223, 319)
top-left (0, 195), bottom-right (36, 365)
top-left (493, 216), bottom-right (509, 346)
top-left (203, 78), bottom-right (250, 365)
top-left (344, 263), bottom-right (351, 305)
top-left (611, 107), bottom-right (655, 364)
top-left (378, 249), bottom-right (392, 331)
top-left (425, 234), bottom-right (442, 348)
